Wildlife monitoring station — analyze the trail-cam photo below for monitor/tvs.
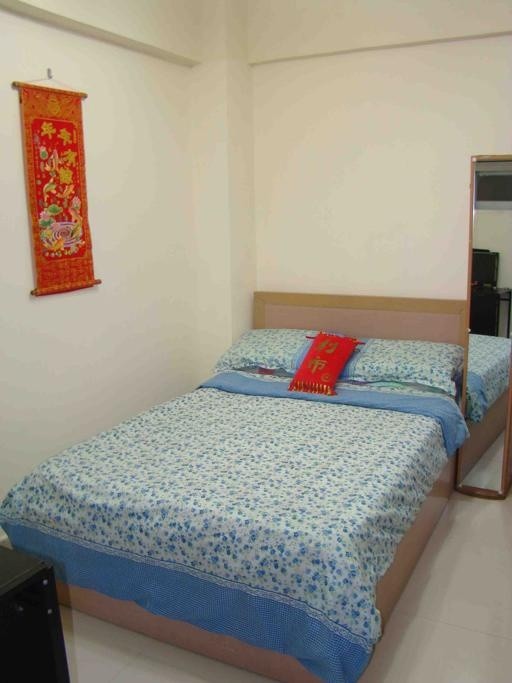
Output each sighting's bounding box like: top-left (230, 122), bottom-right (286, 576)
top-left (471, 251), bottom-right (499, 291)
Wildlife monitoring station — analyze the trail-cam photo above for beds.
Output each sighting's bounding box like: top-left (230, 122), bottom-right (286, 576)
top-left (458, 328), bottom-right (511, 485)
top-left (0, 288), bottom-right (468, 683)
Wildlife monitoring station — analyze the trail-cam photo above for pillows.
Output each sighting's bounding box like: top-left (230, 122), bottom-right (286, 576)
top-left (212, 326), bottom-right (464, 398)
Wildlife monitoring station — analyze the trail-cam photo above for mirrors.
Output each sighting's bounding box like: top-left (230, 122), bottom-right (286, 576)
top-left (453, 151), bottom-right (511, 498)
